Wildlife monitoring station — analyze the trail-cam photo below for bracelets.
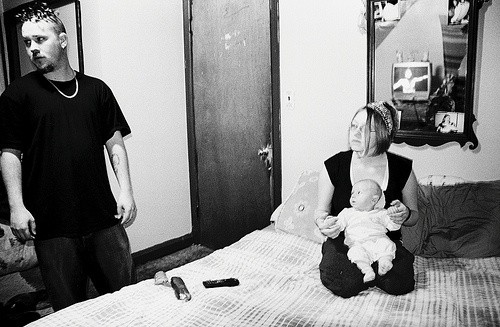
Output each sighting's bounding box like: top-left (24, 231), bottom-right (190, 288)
top-left (404, 206), bottom-right (411, 222)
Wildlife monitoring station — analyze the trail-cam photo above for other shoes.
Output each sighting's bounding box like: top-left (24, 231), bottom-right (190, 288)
top-left (5, 290), bottom-right (50, 313)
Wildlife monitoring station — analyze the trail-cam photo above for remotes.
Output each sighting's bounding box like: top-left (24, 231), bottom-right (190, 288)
top-left (202, 278), bottom-right (239, 288)
top-left (171, 277), bottom-right (191, 300)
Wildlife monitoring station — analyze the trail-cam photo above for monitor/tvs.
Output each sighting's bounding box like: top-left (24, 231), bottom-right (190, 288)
top-left (391, 62), bottom-right (431, 101)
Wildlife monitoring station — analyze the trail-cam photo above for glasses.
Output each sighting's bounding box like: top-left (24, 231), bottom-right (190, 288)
top-left (351, 121), bottom-right (375, 132)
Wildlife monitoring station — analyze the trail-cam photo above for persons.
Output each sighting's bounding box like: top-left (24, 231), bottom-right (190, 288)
top-left (448, 0), bottom-right (470, 24)
top-left (436, 114), bottom-right (457, 133)
top-left (0, 0), bottom-right (137, 313)
top-left (394, 68), bottom-right (428, 93)
top-left (314, 100), bottom-right (419, 298)
top-left (323, 179), bottom-right (402, 283)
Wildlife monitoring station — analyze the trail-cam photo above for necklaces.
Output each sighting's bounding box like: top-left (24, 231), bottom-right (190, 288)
top-left (47, 73), bottom-right (79, 99)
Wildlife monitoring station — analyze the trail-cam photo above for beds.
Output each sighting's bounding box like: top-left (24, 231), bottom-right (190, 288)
top-left (24, 222), bottom-right (500, 327)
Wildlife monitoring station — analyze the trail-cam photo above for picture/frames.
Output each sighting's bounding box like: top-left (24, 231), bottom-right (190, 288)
top-left (11, 0), bottom-right (84, 78)
top-left (366, 0), bottom-right (489, 149)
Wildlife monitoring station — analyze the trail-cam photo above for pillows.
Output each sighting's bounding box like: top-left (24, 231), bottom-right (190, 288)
top-left (401, 180), bottom-right (500, 258)
top-left (270, 171), bottom-right (327, 244)
